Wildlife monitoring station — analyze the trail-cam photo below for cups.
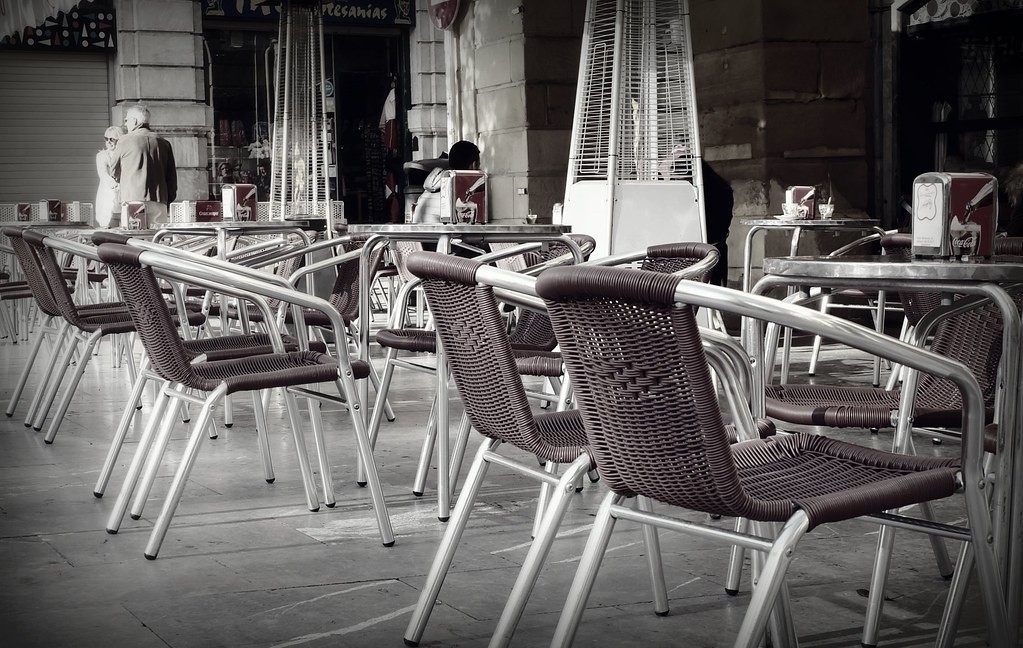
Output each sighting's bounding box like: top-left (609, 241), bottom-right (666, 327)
top-left (819, 204), bottom-right (835, 220)
top-left (782, 203), bottom-right (806, 218)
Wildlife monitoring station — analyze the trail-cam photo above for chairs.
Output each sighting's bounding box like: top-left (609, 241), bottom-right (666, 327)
top-left (3, 217), bottom-right (1021, 648)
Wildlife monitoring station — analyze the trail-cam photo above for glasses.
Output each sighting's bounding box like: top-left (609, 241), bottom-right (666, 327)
top-left (124, 118), bottom-right (127, 123)
top-left (104, 136), bottom-right (118, 142)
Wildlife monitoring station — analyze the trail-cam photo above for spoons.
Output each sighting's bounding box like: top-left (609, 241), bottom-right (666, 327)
top-left (824, 196), bottom-right (831, 216)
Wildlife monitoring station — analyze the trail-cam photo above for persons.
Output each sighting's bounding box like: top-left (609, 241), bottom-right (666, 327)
top-left (109, 106), bottom-right (178, 229)
top-left (412, 141), bottom-right (492, 261)
top-left (258, 164), bottom-right (271, 189)
top-left (218, 162), bottom-right (236, 179)
top-left (629, 109), bottom-right (732, 285)
top-left (96, 127), bottom-right (124, 228)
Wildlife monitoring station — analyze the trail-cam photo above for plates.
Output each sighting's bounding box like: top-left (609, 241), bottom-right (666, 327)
top-left (773, 215), bottom-right (802, 221)
